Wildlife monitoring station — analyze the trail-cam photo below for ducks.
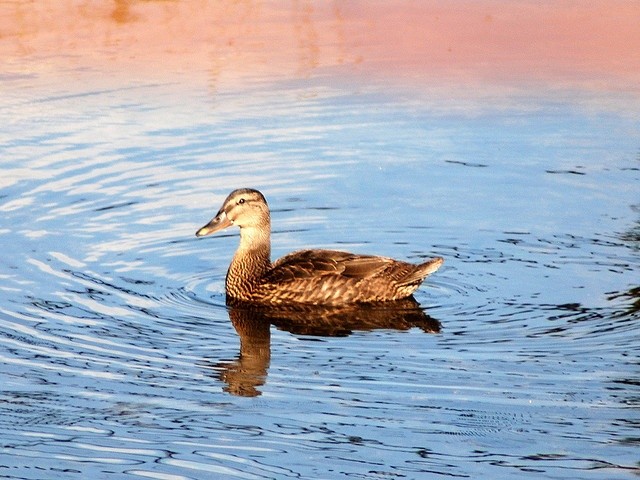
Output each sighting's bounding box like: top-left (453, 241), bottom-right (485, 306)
top-left (194, 186), bottom-right (445, 310)
